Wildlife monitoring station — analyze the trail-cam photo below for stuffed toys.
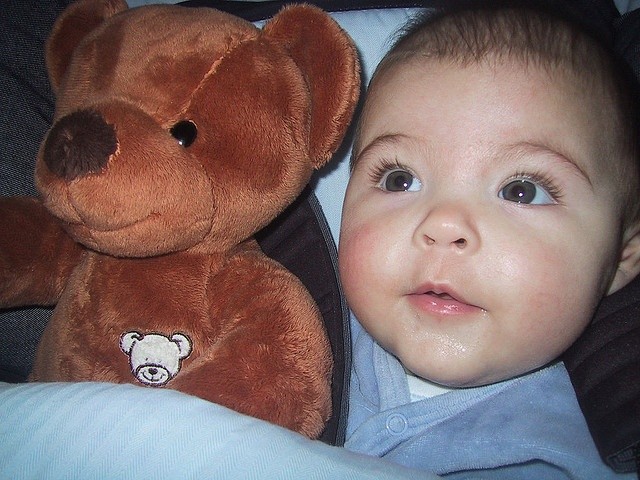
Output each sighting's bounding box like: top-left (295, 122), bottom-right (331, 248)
top-left (0, 0), bottom-right (362, 440)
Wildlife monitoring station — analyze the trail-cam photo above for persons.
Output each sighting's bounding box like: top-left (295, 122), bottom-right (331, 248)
top-left (338, 2), bottom-right (640, 480)
